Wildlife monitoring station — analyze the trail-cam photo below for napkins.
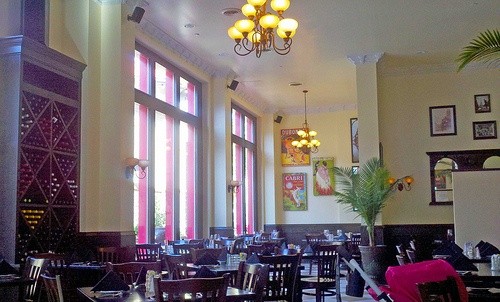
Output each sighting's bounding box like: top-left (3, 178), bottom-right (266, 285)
top-left (194, 266), bottom-right (216, 278)
top-left (136, 265), bottom-right (147, 285)
top-left (475, 240), bottom-right (500, 257)
top-left (90, 270), bottom-right (130, 292)
top-left (246, 251), bottom-right (263, 263)
top-left (446, 252), bottom-right (478, 271)
top-left (194, 249), bottom-right (221, 266)
top-left (0, 259), bottom-right (18, 275)
top-left (446, 244), bottom-right (465, 255)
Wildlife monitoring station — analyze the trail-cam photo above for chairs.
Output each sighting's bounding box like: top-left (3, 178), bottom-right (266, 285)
top-left (0, 226), bottom-right (361, 302)
top-left (395, 239), bottom-right (419, 265)
top-left (418, 276), bottom-right (463, 302)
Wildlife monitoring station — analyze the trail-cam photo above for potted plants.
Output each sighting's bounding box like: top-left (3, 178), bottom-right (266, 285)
top-left (332, 157), bottom-right (414, 277)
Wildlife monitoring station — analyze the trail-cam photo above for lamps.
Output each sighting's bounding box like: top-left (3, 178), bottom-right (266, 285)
top-left (226, 80), bottom-right (239, 92)
top-left (227, 0), bottom-right (298, 59)
top-left (227, 181), bottom-right (242, 194)
top-left (125, 159), bottom-right (149, 180)
top-left (292, 90), bottom-right (322, 152)
top-left (274, 116), bottom-right (283, 124)
top-left (127, 7), bottom-right (145, 23)
top-left (387, 176), bottom-right (413, 191)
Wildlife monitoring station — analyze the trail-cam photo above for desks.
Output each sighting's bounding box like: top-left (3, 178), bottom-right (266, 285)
top-left (48, 263), bottom-right (104, 286)
top-left (302, 239), bottom-right (345, 268)
top-left (178, 261), bottom-right (273, 278)
top-left (458, 261), bottom-right (500, 302)
top-left (302, 252), bottom-right (336, 276)
top-left (75, 284), bottom-right (256, 302)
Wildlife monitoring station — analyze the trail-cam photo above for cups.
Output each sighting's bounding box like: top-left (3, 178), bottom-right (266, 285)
top-left (329, 234), bottom-right (333, 242)
top-left (337, 230), bottom-right (342, 236)
top-left (214, 234), bottom-right (220, 240)
top-left (240, 252), bottom-right (247, 261)
top-left (491, 254), bottom-right (500, 270)
top-left (324, 230), bottom-right (329, 237)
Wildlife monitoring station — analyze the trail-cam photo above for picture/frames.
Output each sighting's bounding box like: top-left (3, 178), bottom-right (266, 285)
top-left (429, 105), bottom-right (457, 137)
top-left (474, 93), bottom-right (491, 113)
top-left (379, 142), bottom-right (383, 164)
top-left (472, 119), bottom-right (497, 141)
